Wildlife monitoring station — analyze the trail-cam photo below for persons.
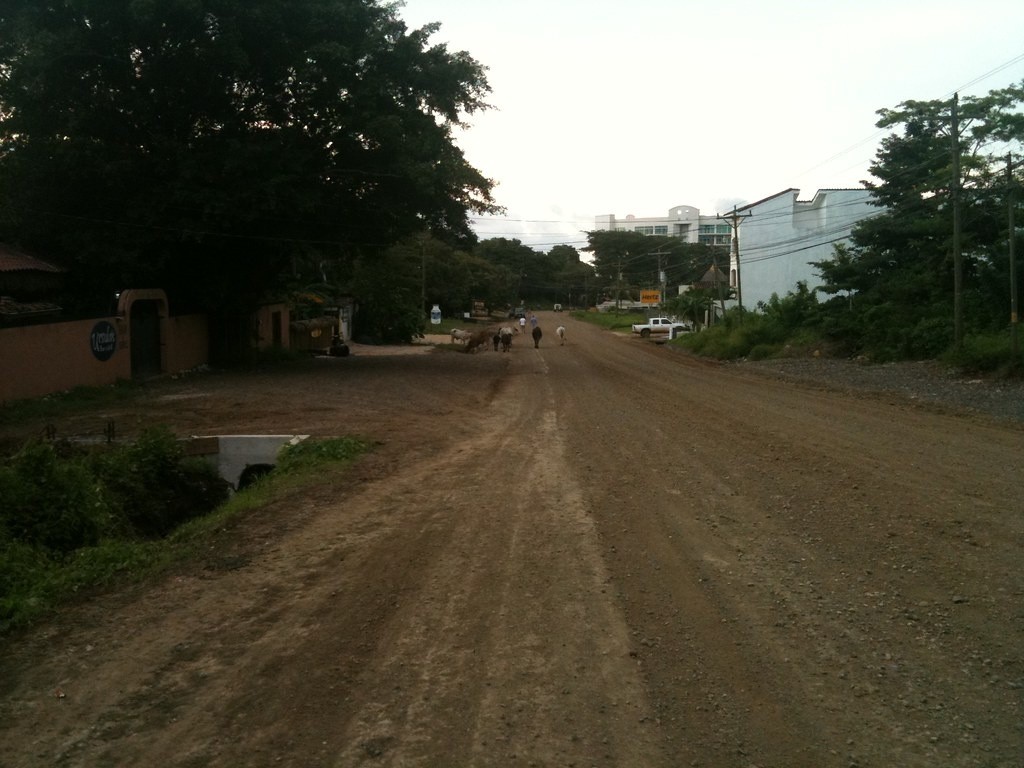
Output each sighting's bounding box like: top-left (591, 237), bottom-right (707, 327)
top-left (519, 315), bottom-right (526, 335)
top-left (664, 315), bottom-right (668, 318)
top-left (672, 314), bottom-right (677, 321)
top-left (530, 314), bottom-right (537, 330)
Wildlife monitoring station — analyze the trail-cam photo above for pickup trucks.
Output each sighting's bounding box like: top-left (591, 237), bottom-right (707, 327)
top-left (632, 318), bottom-right (690, 337)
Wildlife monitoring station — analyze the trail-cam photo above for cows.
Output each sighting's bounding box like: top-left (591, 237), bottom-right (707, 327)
top-left (449, 328), bottom-right (473, 345)
top-left (479, 329), bottom-right (490, 346)
top-left (556, 325), bottom-right (566, 346)
top-left (531, 326), bottom-right (543, 348)
top-left (500, 327), bottom-right (513, 353)
